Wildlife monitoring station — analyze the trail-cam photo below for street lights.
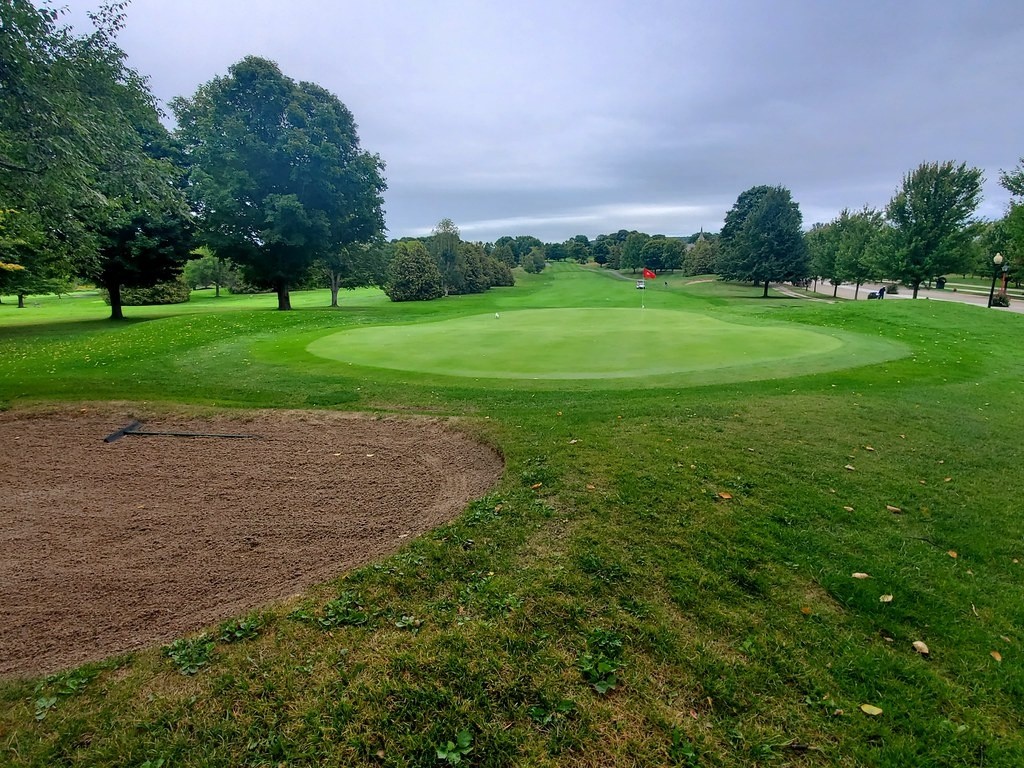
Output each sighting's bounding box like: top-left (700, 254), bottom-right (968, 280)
top-left (988, 253), bottom-right (1003, 308)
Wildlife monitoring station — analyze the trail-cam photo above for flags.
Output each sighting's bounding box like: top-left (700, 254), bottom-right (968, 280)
top-left (644, 268), bottom-right (656, 279)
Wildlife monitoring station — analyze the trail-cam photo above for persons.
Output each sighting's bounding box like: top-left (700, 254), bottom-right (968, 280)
top-left (878, 286), bottom-right (886, 300)
